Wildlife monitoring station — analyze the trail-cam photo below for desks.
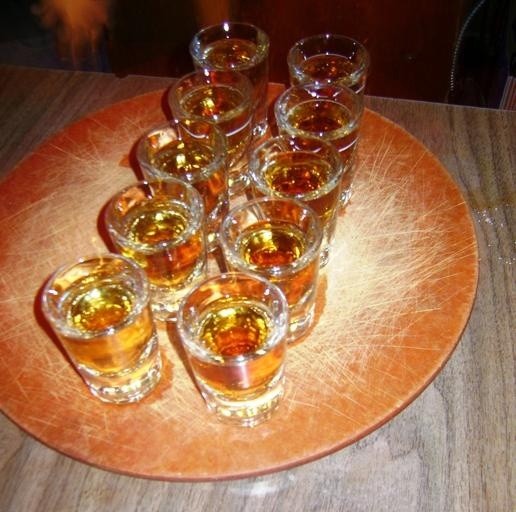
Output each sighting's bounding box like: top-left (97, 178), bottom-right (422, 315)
top-left (0, 64), bottom-right (516, 511)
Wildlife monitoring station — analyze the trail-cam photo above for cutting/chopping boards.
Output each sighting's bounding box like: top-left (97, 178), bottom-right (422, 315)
top-left (0, 80), bottom-right (479, 482)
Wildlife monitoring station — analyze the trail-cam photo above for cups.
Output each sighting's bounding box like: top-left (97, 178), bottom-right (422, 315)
top-left (246, 132), bottom-right (346, 266)
top-left (100, 176), bottom-right (207, 321)
top-left (171, 69), bottom-right (259, 193)
top-left (275, 80), bottom-right (362, 205)
top-left (177, 271), bottom-right (289, 426)
top-left (286, 34), bottom-right (368, 95)
top-left (219, 198), bottom-right (323, 341)
top-left (135, 117), bottom-right (231, 254)
top-left (189, 22), bottom-right (272, 141)
top-left (43, 254), bottom-right (166, 405)
